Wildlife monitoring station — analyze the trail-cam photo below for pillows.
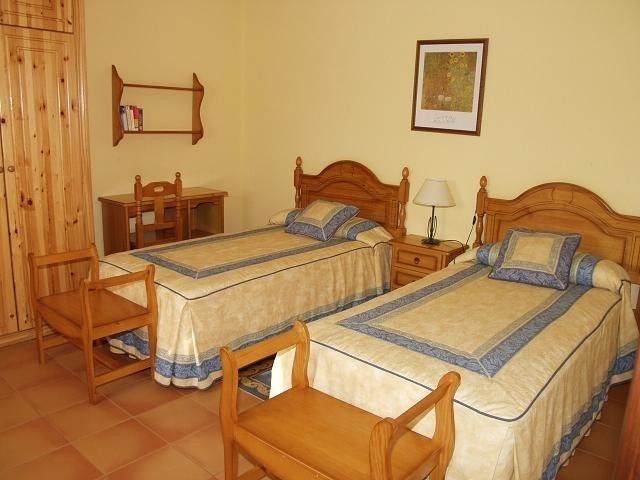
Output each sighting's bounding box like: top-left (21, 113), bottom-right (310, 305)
top-left (487, 226), bottom-right (582, 291)
top-left (284, 199), bottom-right (360, 242)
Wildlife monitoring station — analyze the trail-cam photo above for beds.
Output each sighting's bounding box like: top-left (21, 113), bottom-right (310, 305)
top-left (276, 175), bottom-right (640, 480)
top-left (99, 156), bottom-right (410, 388)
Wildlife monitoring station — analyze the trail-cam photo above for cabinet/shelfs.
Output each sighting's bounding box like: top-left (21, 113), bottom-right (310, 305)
top-left (219, 319), bottom-right (462, 480)
top-left (110, 64), bottom-right (206, 147)
top-left (27, 242), bottom-right (159, 405)
top-left (0, 0), bottom-right (95, 349)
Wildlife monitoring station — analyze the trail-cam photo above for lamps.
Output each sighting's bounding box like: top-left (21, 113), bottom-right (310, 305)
top-left (412, 178), bottom-right (457, 245)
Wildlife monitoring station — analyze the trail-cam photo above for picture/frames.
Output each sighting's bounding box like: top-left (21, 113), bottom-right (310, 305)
top-left (411, 38), bottom-right (489, 137)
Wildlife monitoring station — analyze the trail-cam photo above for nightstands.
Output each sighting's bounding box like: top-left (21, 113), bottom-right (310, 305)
top-left (387, 233), bottom-right (470, 292)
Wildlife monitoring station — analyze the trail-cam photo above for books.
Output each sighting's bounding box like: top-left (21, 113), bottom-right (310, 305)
top-left (120, 104), bottom-right (143, 131)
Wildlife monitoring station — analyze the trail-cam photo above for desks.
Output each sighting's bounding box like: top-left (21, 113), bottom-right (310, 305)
top-left (97, 186), bottom-right (228, 256)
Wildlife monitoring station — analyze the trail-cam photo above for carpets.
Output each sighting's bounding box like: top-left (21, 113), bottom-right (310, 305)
top-left (237, 353), bottom-right (277, 401)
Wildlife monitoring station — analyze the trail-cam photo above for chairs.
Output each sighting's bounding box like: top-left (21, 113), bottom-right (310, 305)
top-left (129, 172), bottom-right (183, 249)
